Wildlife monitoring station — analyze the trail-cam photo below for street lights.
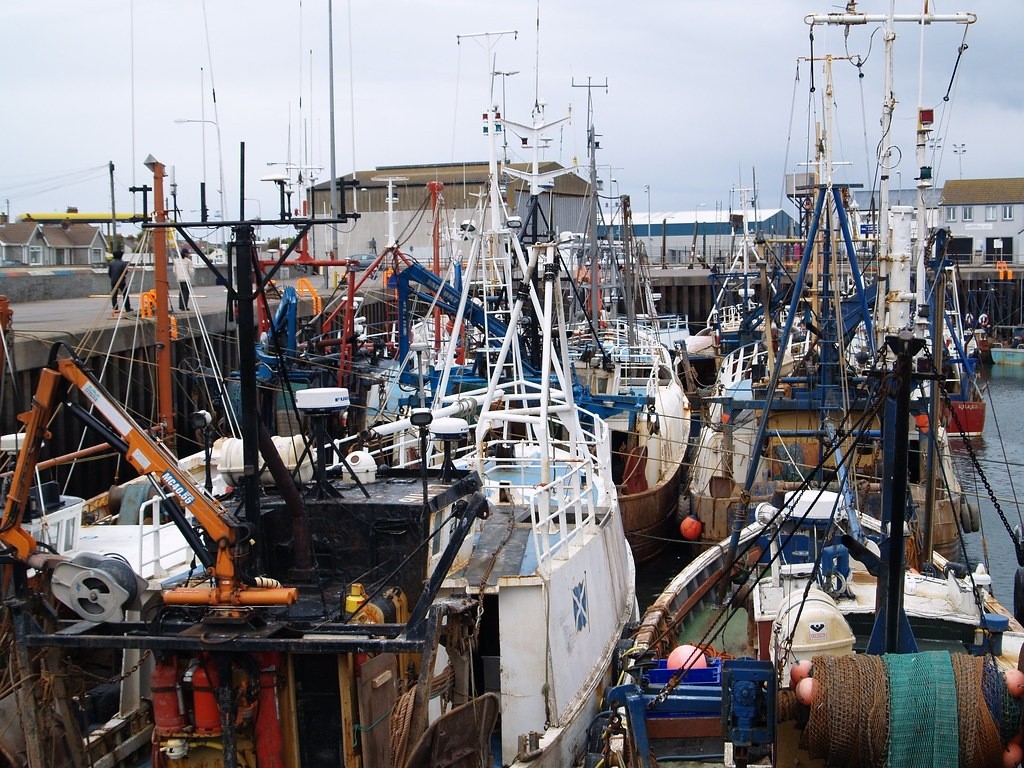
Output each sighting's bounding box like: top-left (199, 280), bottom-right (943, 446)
top-left (642, 183), bottom-right (650, 240)
top-left (952, 143), bottom-right (968, 179)
top-left (611, 177), bottom-right (622, 246)
top-left (172, 119), bottom-right (225, 253)
top-left (695, 203), bottom-right (709, 223)
top-left (244, 198), bottom-right (261, 239)
top-left (896, 171), bottom-right (902, 206)
top-left (266, 162), bottom-right (302, 217)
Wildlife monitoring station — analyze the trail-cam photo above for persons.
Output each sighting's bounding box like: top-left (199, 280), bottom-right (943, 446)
top-left (172, 248), bottom-right (194, 311)
top-left (108, 249), bottom-right (134, 312)
top-left (371, 237), bottom-right (377, 253)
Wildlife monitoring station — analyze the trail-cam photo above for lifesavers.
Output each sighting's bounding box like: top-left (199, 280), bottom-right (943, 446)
top-left (979, 313), bottom-right (989, 326)
top-left (965, 314), bottom-right (973, 324)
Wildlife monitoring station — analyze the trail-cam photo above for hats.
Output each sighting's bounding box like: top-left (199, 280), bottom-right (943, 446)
top-left (181, 248), bottom-right (191, 254)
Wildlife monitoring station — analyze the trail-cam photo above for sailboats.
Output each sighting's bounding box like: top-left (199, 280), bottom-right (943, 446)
top-left (1, 3), bottom-right (1024, 767)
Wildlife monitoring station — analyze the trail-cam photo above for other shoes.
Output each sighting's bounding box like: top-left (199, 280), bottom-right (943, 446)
top-left (125, 308), bottom-right (134, 312)
top-left (182, 308), bottom-right (190, 311)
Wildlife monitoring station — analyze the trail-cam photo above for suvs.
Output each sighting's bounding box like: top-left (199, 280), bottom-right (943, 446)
top-left (345, 253), bottom-right (389, 271)
top-left (584, 252), bottom-right (636, 270)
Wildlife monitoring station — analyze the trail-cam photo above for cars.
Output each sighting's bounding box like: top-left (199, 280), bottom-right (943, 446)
top-left (3, 259), bottom-right (21, 267)
